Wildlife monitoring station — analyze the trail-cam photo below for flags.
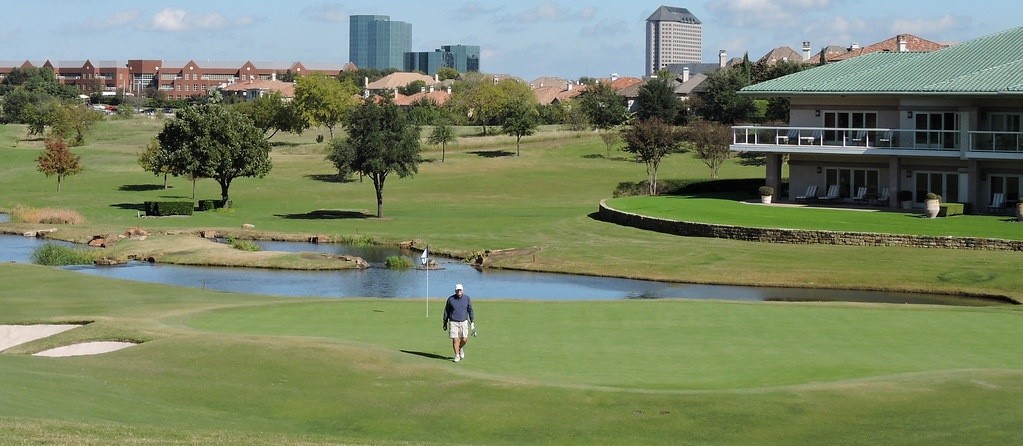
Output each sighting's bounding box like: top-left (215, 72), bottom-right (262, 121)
top-left (420, 247), bottom-right (427, 265)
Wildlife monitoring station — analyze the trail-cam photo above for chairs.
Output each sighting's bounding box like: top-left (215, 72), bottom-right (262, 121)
top-left (852, 131), bottom-right (866, 145)
top-left (879, 131), bottom-right (894, 146)
top-left (818, 185), bottom-right (841, 206)
top-left (800, 130), bottom-right (821, 145)
top-left (877, 187), bottom-right (890, 207)
top-left (775, 129), bottom-right (798, 145)
top-left (853, 186), bottom-right (868, 207)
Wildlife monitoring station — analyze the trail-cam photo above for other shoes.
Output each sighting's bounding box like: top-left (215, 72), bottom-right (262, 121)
top-left (453, 355), bottom-right (460, 363)
top-left (459, 345), bottom-right (464, 359)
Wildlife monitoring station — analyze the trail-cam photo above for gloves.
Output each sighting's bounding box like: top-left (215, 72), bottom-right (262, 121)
top-left (443, 321), bottom-right (447, 331)
top-left (470, 321), bottom-right (474, 330)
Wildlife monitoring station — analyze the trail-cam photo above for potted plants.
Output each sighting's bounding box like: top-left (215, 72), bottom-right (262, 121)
top-left (759, 186), bottom-right (774, 203)
top-left (1015, 198), bottom-right (1023, 221)
top-left (899, 190), bottom-right (943, 219)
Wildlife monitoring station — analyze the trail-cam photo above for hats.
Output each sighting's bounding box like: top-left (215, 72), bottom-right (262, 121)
top-left (455, 284), bottom-right (463, 291)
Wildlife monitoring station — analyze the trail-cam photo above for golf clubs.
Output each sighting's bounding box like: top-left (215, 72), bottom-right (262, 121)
top-left (468, 320), bottom-right (478, 337)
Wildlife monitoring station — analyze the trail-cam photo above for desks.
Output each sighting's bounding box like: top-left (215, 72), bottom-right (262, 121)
top-left (863, 194), bottom-right (878, 208)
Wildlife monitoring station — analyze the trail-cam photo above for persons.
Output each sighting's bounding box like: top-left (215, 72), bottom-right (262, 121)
top-left (443, 283), bottom-right (476, 362)
